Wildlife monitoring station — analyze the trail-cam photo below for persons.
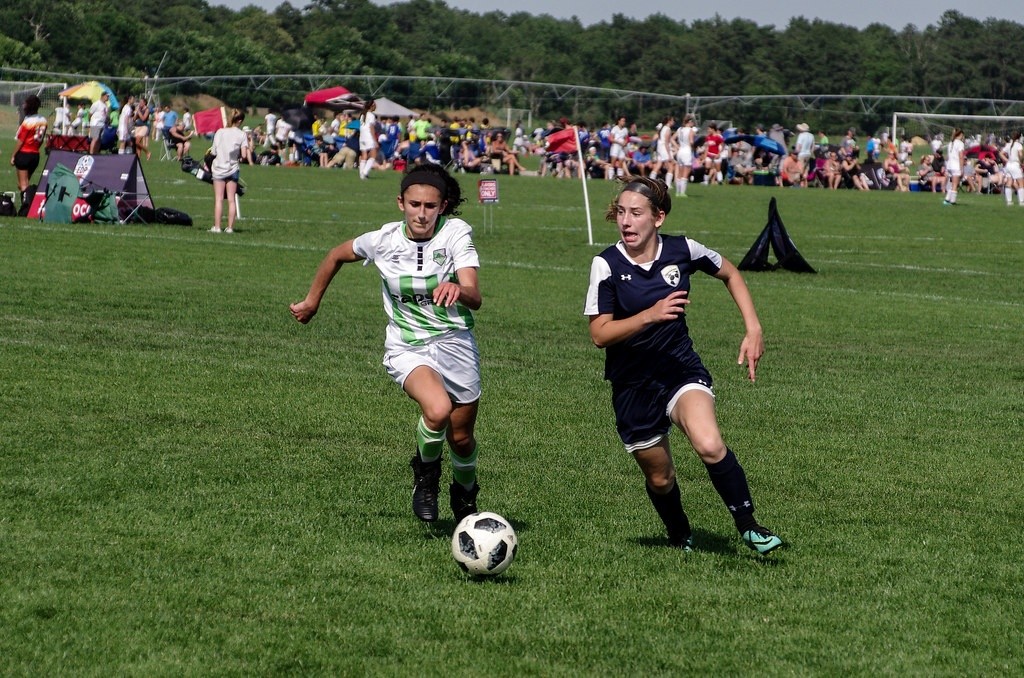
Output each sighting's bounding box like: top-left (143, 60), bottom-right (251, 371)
top-left (10, 94), bottom-right (49, 190)
top-left (108, 109), bottom-right (119, 127)
top-left (151, 105), bottom-right (194, 161)
top-left (128, 98), bottom-right (152, 161)
top-left (72, 104), bottom-right (83, 128)
top-left (808, 125), bottom-right (1024, 208)
top-left (289, 164), bottom-right (484, 522)
top-left (244, 107), bottom-right (329, 167)
top-left (62, 104), bottom-right (71, 135)
top-left (88, 92), bottom-right (109, 155)
top-left (355, 99), bottom-right (380, 179)
top-left (210, 109), bottom-right (249, 233)
top-left (606, 115), bottom-right (812, 197)
top-left (489, 120), bottom-right (613, 181)
top-left (328, 113), bottom-right (492, 171)
top-left (116, 94), bottom-right (135, 154)
top-left (583, 176), bottom-right (784, 556)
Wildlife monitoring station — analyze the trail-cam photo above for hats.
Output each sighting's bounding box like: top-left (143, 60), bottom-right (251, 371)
top-left (102, 91), bottom-right (111, 96)
top-left (797, 123), bottom-right (809, 132)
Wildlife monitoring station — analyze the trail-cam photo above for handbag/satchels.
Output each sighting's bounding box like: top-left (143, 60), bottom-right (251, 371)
top-left (0, 192), bottom-right (16, 217)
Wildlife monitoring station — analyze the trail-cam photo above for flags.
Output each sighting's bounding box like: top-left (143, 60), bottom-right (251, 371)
top-left (191, 107), bottom-right (224, 132)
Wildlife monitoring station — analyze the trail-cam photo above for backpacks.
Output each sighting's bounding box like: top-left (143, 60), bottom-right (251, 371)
top-left (18, 184), bottom-right (39, 217)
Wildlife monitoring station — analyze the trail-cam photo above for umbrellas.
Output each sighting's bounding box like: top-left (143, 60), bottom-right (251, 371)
top-left (721, 132), bottom-right (785, 157)
top-left (56, 81), bottom-right (119, 110)
top-left (373, 96), bottom-right (419, 118)
top-left (304, 85), bottom-right (365, 117)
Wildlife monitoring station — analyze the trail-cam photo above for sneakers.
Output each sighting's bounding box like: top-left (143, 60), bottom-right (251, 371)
top-left (449, 483), bottom-right (481, 524)
top-left (743, 525), bottom-right (782, 554)
top-left (679, 535), bottom-right (695, 558)
top-left (409, 456), bottom-right (442, 521)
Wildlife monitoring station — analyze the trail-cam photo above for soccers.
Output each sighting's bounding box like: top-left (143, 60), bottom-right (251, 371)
top-left (452, 511), bottom-right (516, 577)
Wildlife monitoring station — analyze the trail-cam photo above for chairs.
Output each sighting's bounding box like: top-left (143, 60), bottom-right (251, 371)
top-left (159, 125), bottom-right (186, 162)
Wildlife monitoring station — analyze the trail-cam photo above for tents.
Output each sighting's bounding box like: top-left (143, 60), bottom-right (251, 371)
top-left (734, 197), bottom-right (817, 274)
top-left (26, 148), bottom-right (155, 227)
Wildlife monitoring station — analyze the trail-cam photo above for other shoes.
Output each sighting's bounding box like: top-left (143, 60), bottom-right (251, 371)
top-left (224, 227), bottom-right (233, 234)
top-left (676, 192), bottom-right (688, 198)
top-left (208, 225), bottom-right (222, 234)
top-left (360, 173), bottom-right (369, 181)
top-left (943, 199), bottom-right (956, 206)
